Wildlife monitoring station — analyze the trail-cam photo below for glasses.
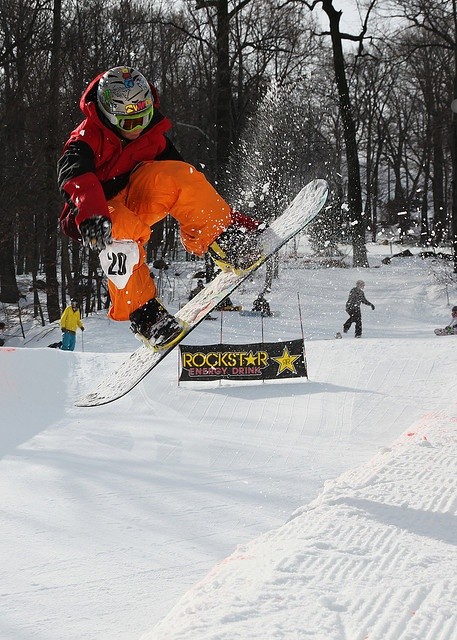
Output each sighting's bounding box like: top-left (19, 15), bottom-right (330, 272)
top-left (98, 99), bottom-right (154, 132)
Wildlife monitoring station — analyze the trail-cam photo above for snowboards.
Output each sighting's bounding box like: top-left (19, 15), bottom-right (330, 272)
top-left (239, 310), bottom-right (281, 317)
top-left (74, 180), bottom-right (329, 408)
top-left (435, 327), bottom-right (457, 335)
top-left (206, 317), bottom-right (219, 320)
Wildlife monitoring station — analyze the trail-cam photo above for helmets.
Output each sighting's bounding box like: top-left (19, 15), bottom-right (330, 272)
top-left (97, 67), bottom-right (154, 139)
top-left (71, 297), bottom-right (78, 307)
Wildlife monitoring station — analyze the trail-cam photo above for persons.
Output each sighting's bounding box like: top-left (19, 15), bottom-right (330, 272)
top-left (433, 306), bottom-right (456, 335)
top-left (59, 299), bottom-right (84, 351)
top-left (57, 67), bottom-right (267, 350)
top-left (252, 293), bottom-right (273, 317)
top-left (189, 280), bottom-right (205, 300)
top-left (342, 279), bottom-right (375, 339)
top-left (217, 297), bottom-right (233, 307)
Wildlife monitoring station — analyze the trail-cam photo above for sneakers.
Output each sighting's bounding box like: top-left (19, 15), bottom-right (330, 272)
top-left (130, 300), bottom-right (185, 347)
top-left (207, 229), bottom-right (263, 272)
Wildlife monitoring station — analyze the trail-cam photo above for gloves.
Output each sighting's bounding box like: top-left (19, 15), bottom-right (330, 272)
top-left (62, 328), bottom-right (65, 332)
top-left (80, 219), bottom-right (113, 251)
top-left (80, 327), bottom-right (84, 330)
